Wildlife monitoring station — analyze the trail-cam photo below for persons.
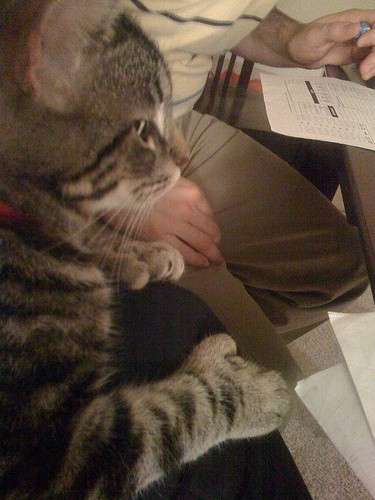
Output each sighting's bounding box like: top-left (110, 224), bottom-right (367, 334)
top-left (103, 1), bottom-right (374, 347)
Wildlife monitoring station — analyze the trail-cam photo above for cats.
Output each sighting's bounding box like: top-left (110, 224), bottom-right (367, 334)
top-left (1, 1), bottom-right (291, 500)
